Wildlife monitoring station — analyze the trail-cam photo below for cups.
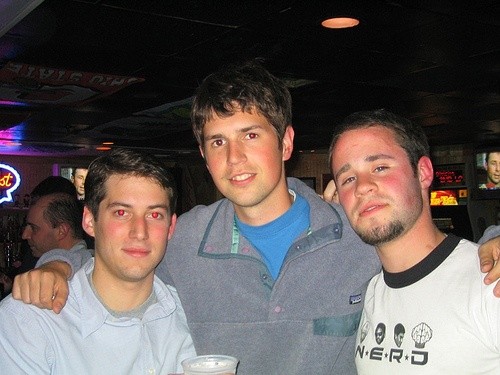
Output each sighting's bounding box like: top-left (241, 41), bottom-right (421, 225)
top-left (181, 354), bottom-right (239, 375)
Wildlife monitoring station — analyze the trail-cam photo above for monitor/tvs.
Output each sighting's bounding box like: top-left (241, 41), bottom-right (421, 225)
top-left (52, 163), bottom-right (88, 201)
top-left (469, 146), bottom-right (500, 200)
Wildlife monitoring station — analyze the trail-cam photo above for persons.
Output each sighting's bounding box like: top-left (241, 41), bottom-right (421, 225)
top-left (329, 107), bottom-right (500, 375)
top-left (0, 148), bottom-right (198, 373)
top-left (29, 175), bottom-right (78, 204)
top-left (22, 191), bottom-right (93, 268)
top-left (478, 151), bottom-right (500, 189)
top-left (324, 178), bottom-right (340, 203)
top-left (71, 168), bottom-right (90, 200)
top-left (0, 216), bottom-right (39, 302)
top-left (11, 64), bottom-right (500, 375)
